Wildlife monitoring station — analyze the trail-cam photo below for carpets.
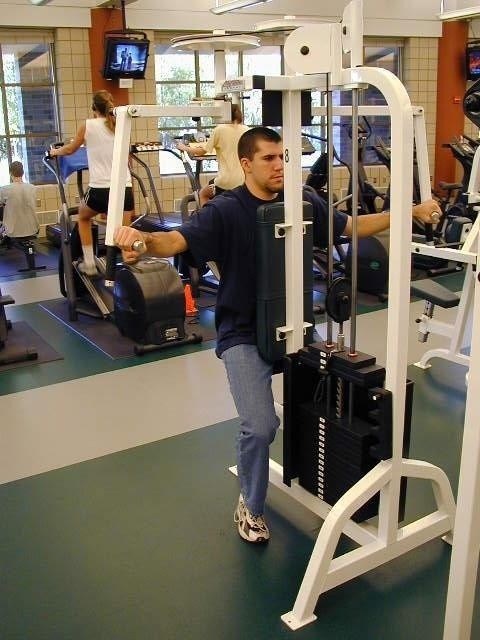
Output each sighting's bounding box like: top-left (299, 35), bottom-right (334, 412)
top-left (1, 321), bottom-right (65, 372)
top-left (0, 247), bottom-right (57, 276)
top-left (314, 278), bottom-right (380, 307)
top-left (37, 298), bottom-right (217, 362)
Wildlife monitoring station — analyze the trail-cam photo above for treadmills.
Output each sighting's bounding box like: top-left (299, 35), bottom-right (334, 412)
top-left (129, 140), bottom-right (195, 232)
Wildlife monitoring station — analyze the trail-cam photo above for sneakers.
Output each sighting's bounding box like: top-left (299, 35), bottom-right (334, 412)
top-left (234, 496), bottom-right (270, 542)
top-left (78, 263), bottom-right (98, 276)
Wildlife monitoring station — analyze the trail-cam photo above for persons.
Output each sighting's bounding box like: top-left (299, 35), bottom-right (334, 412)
top-left (127, 52), bottom-right (132, 71)
top-left (176, 96), bottom-right (253, 207)
top-left (113, 127), bottom-right (443, 545)
top-left (0, 161), bottom-right (40, 242)
top-left (120, 47), bottom-right (128, 70)
top-left (48, 89), bottom-right (139, 276)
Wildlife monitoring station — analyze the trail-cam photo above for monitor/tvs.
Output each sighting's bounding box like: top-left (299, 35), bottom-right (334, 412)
top-left (465, 46), bottom-right (480, 80)
top-left (103, 39), bottom-right (150, 80)
